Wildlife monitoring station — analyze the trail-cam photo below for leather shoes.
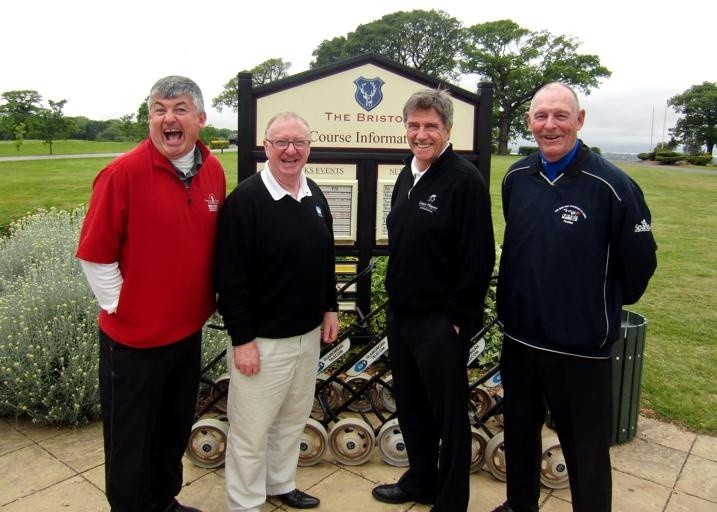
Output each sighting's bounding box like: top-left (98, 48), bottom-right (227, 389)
top-left (269, 489), bottom-right (319, 508)
top-left (491, 501), bottom-right (512, 512)
top-left (163, 497), bottom-right (201, 511)
top-left (373, 484), bottom-right (432, 504)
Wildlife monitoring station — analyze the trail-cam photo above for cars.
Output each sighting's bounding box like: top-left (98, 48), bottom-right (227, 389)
top-left (228, 134), bottom-right (239, 145)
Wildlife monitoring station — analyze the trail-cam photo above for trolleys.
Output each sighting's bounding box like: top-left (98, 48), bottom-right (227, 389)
top-left (184, 258), bottom-right (569, 488)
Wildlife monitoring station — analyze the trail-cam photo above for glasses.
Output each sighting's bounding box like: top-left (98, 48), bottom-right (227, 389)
top-left (266, 138), bottom-right (309, 149)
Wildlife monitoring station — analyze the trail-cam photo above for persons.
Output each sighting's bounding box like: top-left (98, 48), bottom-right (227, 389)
top-left (211, 111), bottom-right (341, 512)
top-left (372, 87), bottom-right (496, 512)
top-left (75, 74), bottom-right (226, 512)
top-left (489, 82), bottom-right (658, 512)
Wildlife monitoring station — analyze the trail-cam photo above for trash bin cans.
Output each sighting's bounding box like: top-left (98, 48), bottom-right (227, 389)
top-left (546, 310), bottom-right (646, 447)
